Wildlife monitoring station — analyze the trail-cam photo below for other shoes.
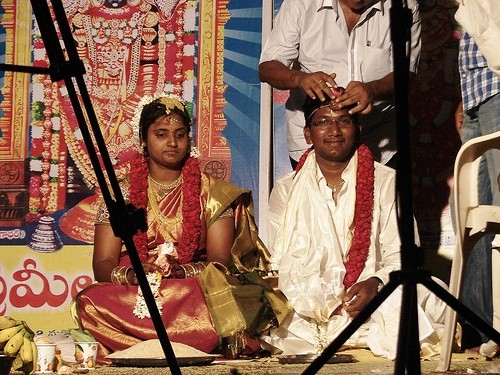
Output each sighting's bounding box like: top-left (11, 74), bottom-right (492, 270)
top-left (478, 339), bottom-right (500, 358)
top-left (451, 328), bottom-right (462, 352)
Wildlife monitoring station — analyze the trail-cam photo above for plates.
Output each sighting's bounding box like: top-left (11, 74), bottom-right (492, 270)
top-left (103, 354), bottom-right (223, 366)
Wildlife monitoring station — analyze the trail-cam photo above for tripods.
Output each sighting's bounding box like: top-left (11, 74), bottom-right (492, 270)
top-left (0, 0), bottom-right (182, 375)
top-left (301, 0), bottom-right (500, 375)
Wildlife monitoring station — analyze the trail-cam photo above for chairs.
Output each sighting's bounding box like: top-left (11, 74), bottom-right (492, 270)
top-left (439, 131), bottom-right (500, 372)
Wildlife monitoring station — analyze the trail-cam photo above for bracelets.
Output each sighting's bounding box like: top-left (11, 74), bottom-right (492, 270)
top-left (111, 265), bottom-right (133, 286)
top-left (179, 262), bottom-right (207, 279)
top-left (371, 277), bottom-right (385, 293)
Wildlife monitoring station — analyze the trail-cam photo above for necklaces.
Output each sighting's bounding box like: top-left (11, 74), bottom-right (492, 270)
top-left (148, 173), bottom-right (184, 227)
top-left (327, 181), bottom-right (345, 193)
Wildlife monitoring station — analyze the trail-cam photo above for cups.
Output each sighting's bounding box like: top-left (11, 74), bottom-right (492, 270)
top-left (36, 344), bottom-right (57, 373)
top-left (74, 342), bottom-right (99, 370)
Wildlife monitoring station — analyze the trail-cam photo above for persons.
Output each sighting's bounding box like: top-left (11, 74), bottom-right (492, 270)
top-left (437, 0), bottom-right (500, 362)
top-left (267, 87), bottom-right (462, 361)
top-left (259, 0), bottom-right (421, 249)
top-left (70, 97), bottom-right (264, 366)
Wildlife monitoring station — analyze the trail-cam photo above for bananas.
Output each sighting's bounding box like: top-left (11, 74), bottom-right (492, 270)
top-left (0, 316), bottom-right (38, 374)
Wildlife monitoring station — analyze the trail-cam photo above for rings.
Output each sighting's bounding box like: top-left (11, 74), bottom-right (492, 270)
top-left (317, 79), bottom-right (323, 85)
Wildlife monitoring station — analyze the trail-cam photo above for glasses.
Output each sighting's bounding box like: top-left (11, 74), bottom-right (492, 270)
top-left (309, 117), bottom-right (355, 129)
